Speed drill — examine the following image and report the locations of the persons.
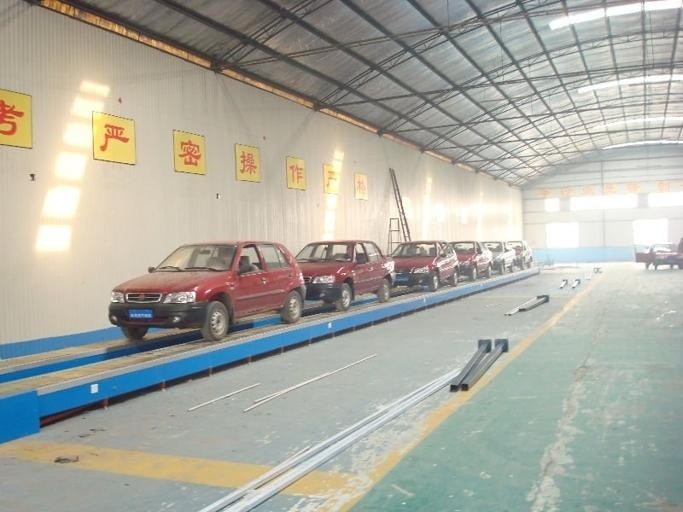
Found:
[678,238,683,269]
[221,247,249,273]
[346,245,364,266]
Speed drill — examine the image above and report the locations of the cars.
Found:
[627,239,682,270]
[382,238,459,293]
[104,237,308,343]
[481,241,517,275]
[445,239,495,283]
[506,239,534,270]
[292,240,397,313]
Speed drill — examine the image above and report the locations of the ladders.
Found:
[389,168,411,242]
